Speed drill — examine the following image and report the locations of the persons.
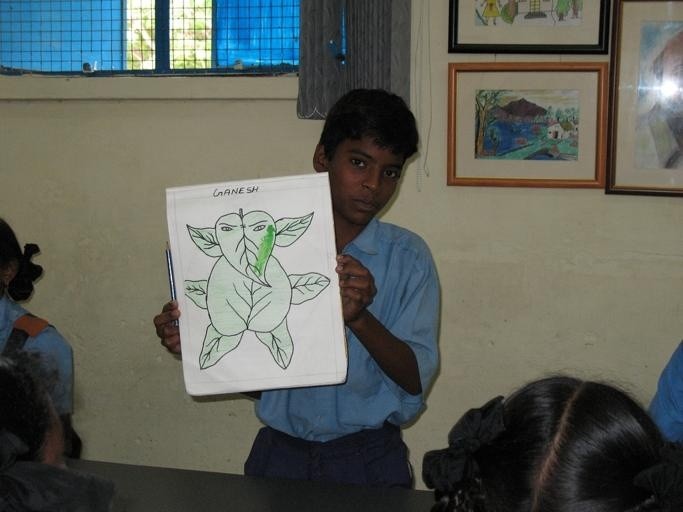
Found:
[0,213,75,469]
[421,370,682,512]
[649,27,682,170]
[0,349,118,512]
[151,85,445,491]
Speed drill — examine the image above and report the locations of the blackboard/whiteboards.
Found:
[166,172,348,396]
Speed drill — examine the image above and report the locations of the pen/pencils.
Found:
[165,242,179,328]
